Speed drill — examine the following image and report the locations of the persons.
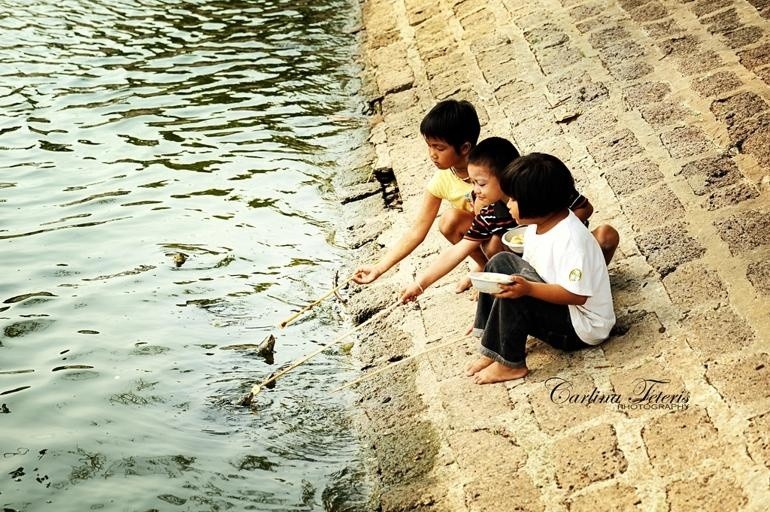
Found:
[464,153,618,386]
[397,133,621,306]
[352,99,491,295]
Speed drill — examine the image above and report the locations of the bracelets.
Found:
[414,279,424,294]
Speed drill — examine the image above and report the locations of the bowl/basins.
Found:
[500,225,530,254]
[466,272,515,295]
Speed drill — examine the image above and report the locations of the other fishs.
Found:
[256,334,275,354]
[230,392,253,406]
